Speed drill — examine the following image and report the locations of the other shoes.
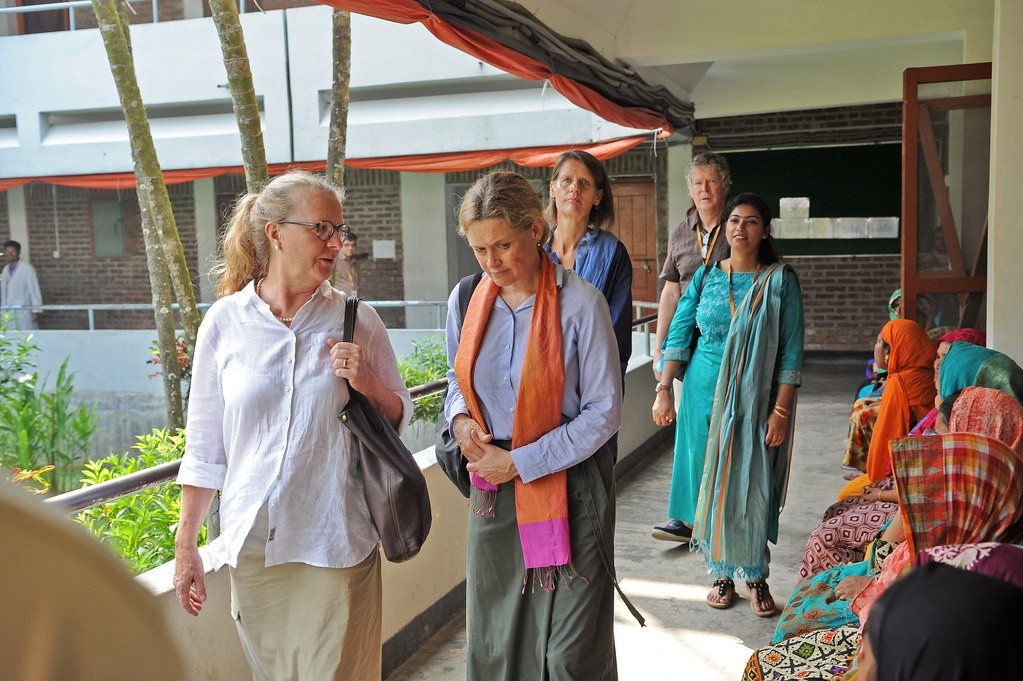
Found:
[845,472,860,480]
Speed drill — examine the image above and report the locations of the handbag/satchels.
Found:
[675,264,714,382]
[433,272,483,498]
[337,296,432,563]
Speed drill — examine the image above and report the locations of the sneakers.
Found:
[652,519,693,542]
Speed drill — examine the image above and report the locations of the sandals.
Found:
[707,577,733,607]
[746,580,778,615]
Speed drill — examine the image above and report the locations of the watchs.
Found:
[655,383,670,392]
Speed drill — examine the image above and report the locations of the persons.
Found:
[543,149,634,464]
[174,171,413,681]
[652,192,805,615]
[651,153,731,542]
[744,318,1023,681]
[333,233,358,297]
[0,240,43,330]
[443,172,622,681]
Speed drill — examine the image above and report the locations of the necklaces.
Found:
[257,277,292,322]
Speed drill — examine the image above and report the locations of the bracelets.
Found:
[774,405,790,418]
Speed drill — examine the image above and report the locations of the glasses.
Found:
[276,221,352,243]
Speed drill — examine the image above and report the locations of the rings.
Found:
[344,359,347,367]
